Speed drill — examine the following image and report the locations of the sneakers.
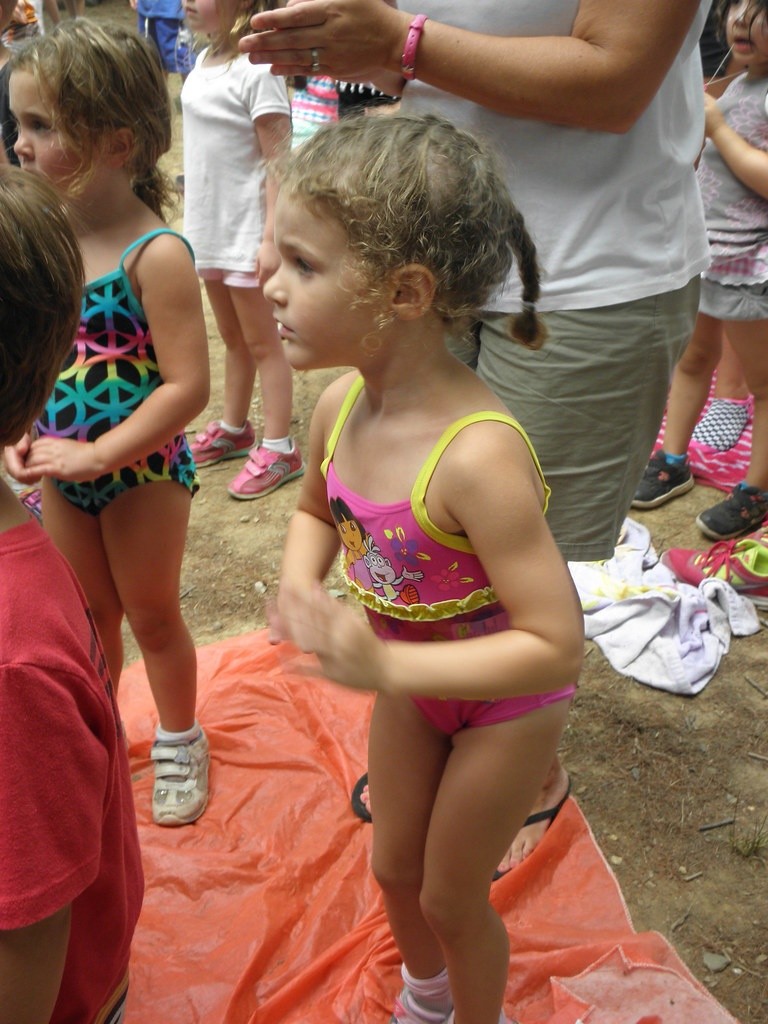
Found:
[228,436,305,501]
[150,732,211,824]
[189,420,255,468]
[659,538,768,610]
[629,450,695,508]
[696,482,768,539]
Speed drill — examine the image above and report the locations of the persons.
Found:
[0,18,209,828]
[0,0,768,880]
[263,116,587,1024]
[0,160,146,1024]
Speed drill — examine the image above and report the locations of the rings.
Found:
[307,47,323,71]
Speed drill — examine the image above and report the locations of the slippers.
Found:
[489,775,572,884]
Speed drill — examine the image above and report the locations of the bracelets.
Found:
[402,12,431,80]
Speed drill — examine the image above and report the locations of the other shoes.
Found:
[350,770,372,822]
